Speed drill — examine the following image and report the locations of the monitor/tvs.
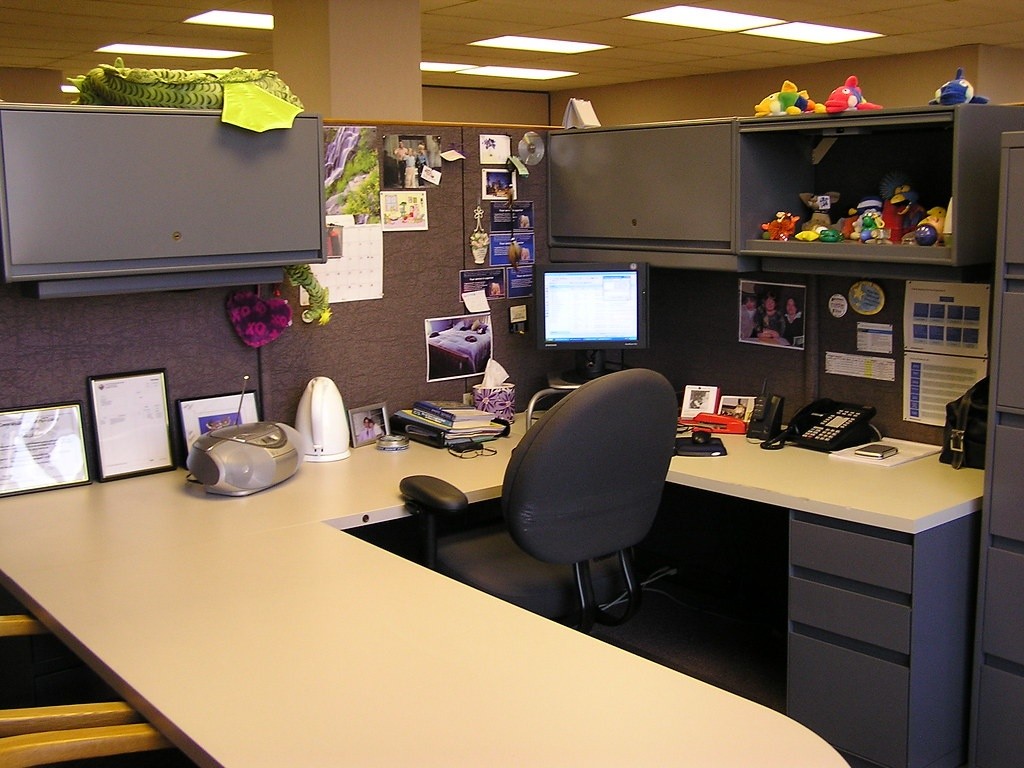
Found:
[534,261,651,389]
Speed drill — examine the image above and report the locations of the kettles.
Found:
[294,376,351,462]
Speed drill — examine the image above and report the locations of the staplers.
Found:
[693,412,749,435]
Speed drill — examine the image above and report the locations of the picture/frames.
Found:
[87,367,179,483]
[175,389,263,471]
[348,403,394,448]
[0,400,94,499]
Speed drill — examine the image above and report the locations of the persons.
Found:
[416,141,430,186]
[783,297,803,347]
[488,182,501,194]
[399,202,407,218]
[403,147,417,188]
[328,224,341,255]
[358,417,377,444]
[394,137,407,185]
[749,296,782,339]
[368,419,385,437]
[504,185,510,195]
[389,206,416,223]
[732,399,746,418]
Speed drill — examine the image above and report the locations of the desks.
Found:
[0,408,985,768]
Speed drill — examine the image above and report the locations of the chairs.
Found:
[400,368,688,631]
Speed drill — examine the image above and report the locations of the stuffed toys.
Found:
[754,80,824,117]
[824,75,883,113]
[926,65,988,108]
[66,52,304,134]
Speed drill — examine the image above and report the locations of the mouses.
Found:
[691,430,711,443]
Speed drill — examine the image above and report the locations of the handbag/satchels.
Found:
[940,376,989,470]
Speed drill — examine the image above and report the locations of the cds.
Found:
[519,131,544,165]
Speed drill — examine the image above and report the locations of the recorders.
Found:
[187,375,306,497]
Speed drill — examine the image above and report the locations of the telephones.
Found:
[785,397,877,451]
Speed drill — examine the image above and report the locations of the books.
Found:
[832,436,943,467]
[394,399,506,433]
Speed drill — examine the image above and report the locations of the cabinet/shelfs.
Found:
[788,515,979,768]
[549,102,1022,274]
[1,113,326,302]
[932,132,1024,768]
